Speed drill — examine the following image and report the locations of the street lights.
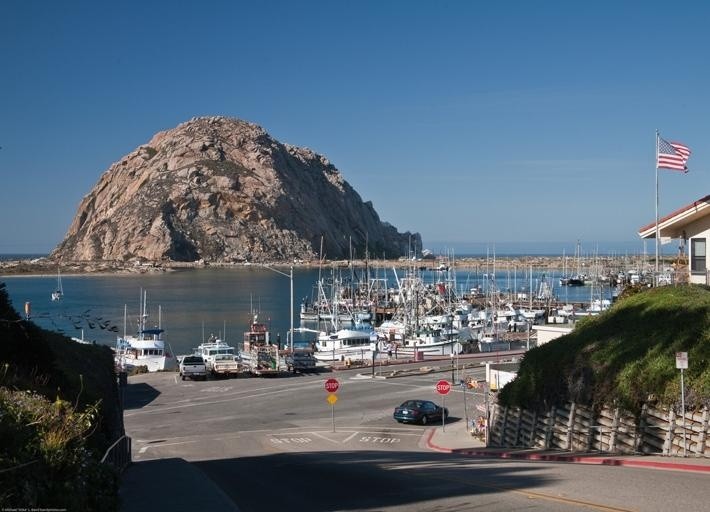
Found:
[244,262,295,353]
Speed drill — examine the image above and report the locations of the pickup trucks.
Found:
[285,351,319,374]
[179,355,207,380]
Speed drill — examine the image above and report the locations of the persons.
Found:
[383,334,398,360]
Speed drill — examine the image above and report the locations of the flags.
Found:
[657,136,691,174]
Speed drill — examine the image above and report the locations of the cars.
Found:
[394,400,448,425]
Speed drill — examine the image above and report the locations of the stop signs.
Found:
[435,380,451,396]
[325,378,339,393]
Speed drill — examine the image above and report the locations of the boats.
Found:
[71,288,178,373]
[196,294,290,380]
[52,267,64,301]
